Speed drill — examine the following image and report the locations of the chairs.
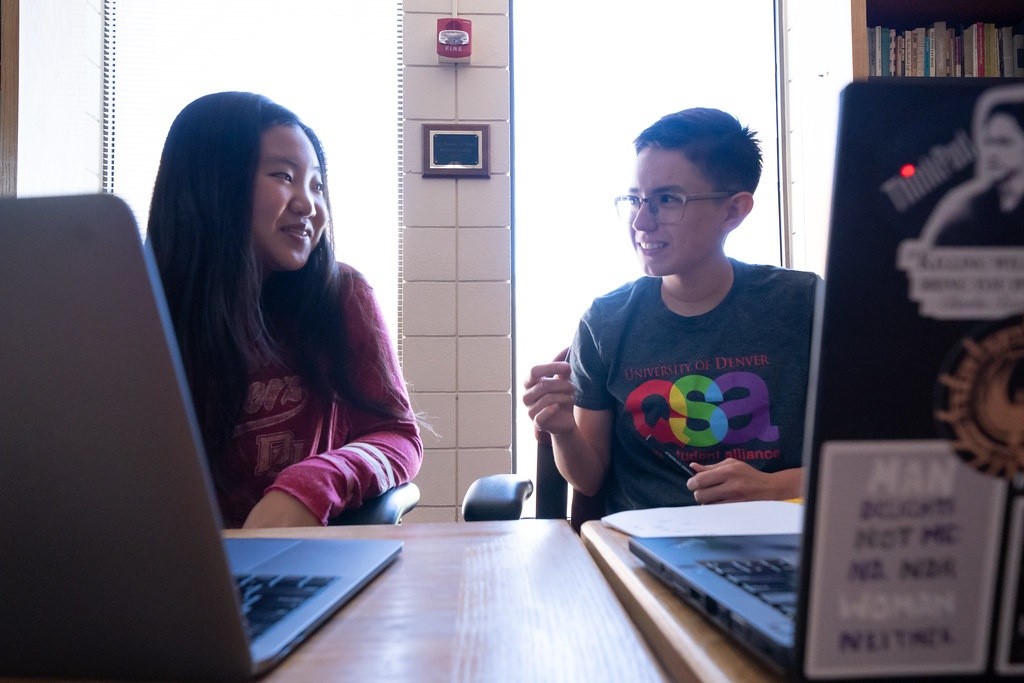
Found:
[462,347,572,520]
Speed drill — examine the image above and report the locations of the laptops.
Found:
[627,77,1024,683]
[2,192,406,683]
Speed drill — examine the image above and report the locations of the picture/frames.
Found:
[421,123,491,179]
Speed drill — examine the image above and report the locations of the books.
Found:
[866,22,1024,78]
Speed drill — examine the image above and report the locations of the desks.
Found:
[222,518,788,682]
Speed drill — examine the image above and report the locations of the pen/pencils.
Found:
[645,433,698,478]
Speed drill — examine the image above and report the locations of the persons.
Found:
[142,89,423,533]
[524,108,818,522]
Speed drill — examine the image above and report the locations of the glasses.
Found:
[614,191,730,224]
